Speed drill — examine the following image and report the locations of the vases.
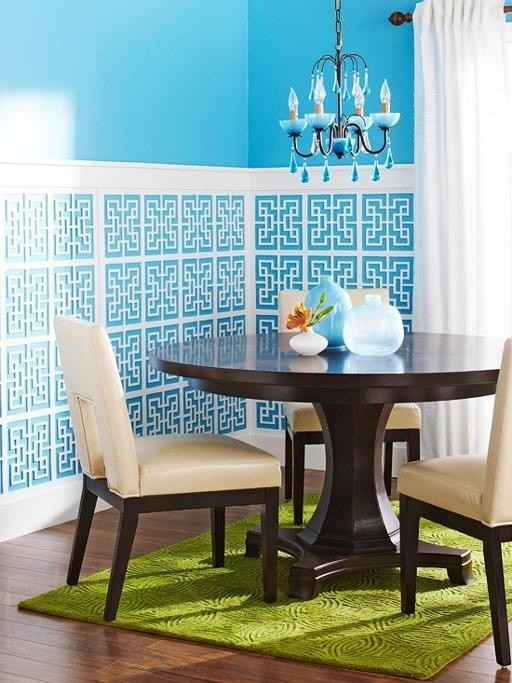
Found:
[304,275,352,346]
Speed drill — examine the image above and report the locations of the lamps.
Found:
[277,0,402,183]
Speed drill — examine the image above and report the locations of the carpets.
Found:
[18,489,511,680]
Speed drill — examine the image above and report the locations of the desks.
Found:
[148,330,510,603]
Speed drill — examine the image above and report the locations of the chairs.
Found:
[50,286,511,666]
